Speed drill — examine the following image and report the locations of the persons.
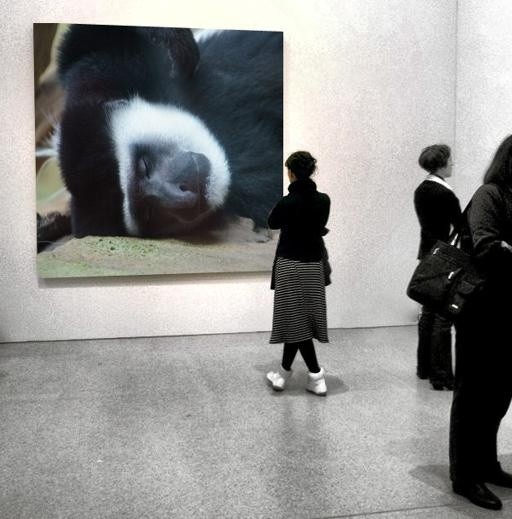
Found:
[413,143,462,392]
[448,134,512,512]
[265,149,331,397]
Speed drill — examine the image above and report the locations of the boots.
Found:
[305,367,328,396]
[265,364,294,392]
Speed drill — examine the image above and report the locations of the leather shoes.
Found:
[452,479,503,511]
[416,367,459,390]
[480,461,512,489]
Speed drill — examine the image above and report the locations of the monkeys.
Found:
[33,24,283,255]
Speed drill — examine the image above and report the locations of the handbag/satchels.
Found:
[407,240,483,325]
